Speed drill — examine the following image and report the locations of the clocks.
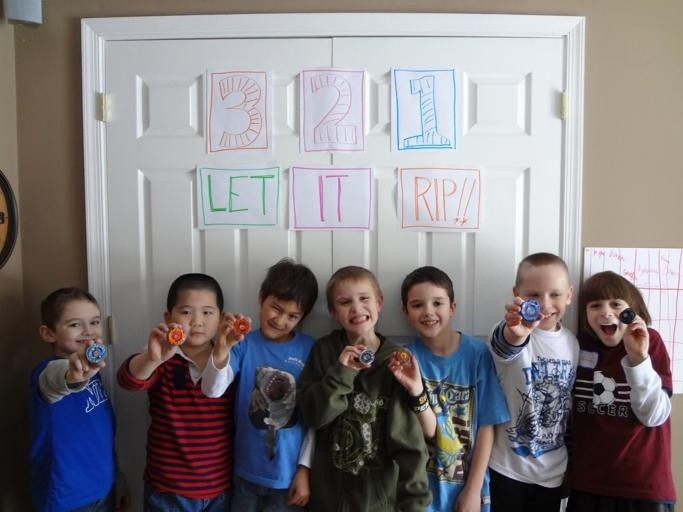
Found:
[0,169,19,269]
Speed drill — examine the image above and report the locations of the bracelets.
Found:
[410,388,430,413]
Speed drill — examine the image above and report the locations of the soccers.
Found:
[592,371,618,410]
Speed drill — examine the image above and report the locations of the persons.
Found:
[201,257,318,512]
[296,266,432,512]
[117,274,235,512]
[565,271,676,512]
[388,267,512,512]
[27,286,115,512]
[486,253,580,512]
